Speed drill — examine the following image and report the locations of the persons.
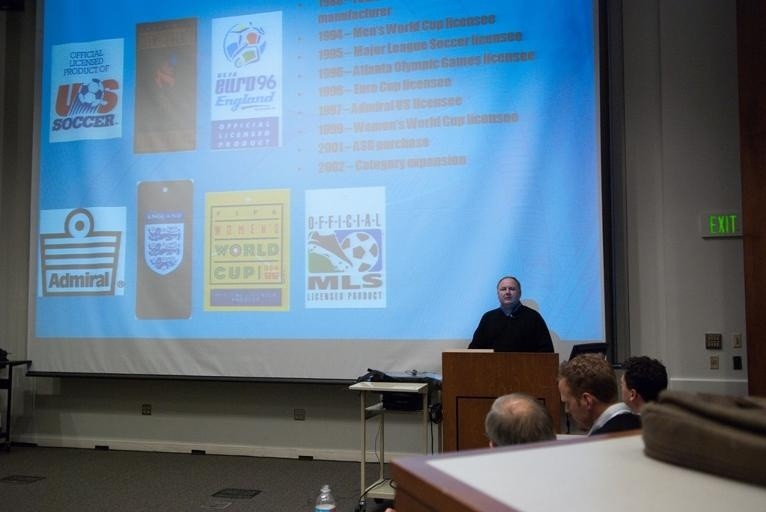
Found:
[619,355,668,417]
[484,392,555,448]
[557,354,641,437]
[467,277,555,353]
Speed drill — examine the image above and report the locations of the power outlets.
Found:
[710,356,719,369]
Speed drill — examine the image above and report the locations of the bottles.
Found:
[314,484,336,512]
